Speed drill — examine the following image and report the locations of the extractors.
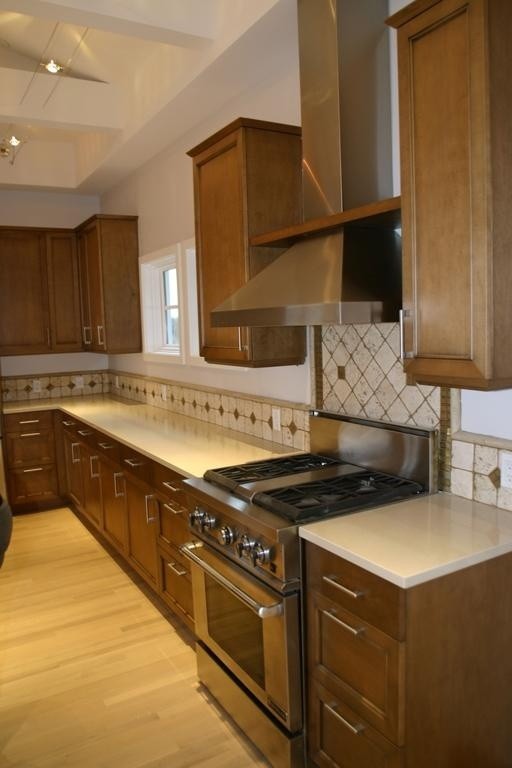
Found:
[207,0,405,328]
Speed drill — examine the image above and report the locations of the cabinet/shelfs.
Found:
[39,221,85,355]
[183,112,310,371]
[0,217,48,358]
[51,402,197,655]
[2,409,61,513]
[295,540,512,768]
[383,0,510,394]
[74,207,144,363]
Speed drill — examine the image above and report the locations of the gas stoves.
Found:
[183,453,423,583]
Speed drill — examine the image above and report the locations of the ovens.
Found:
[176,532,306,768]
